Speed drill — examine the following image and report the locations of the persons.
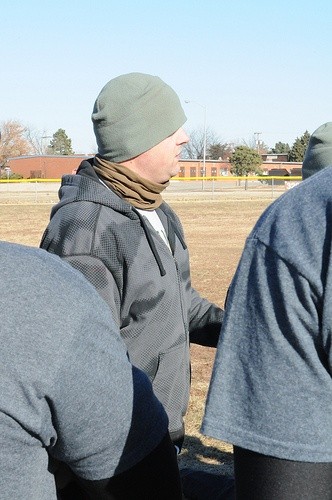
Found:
[0,240,181,500]
[202,161,332,500]
[35,71,232,499]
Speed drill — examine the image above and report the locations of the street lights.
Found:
[185,96,218,189]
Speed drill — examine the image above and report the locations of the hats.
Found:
[301,120,332,180]
[92,73,187,163]
